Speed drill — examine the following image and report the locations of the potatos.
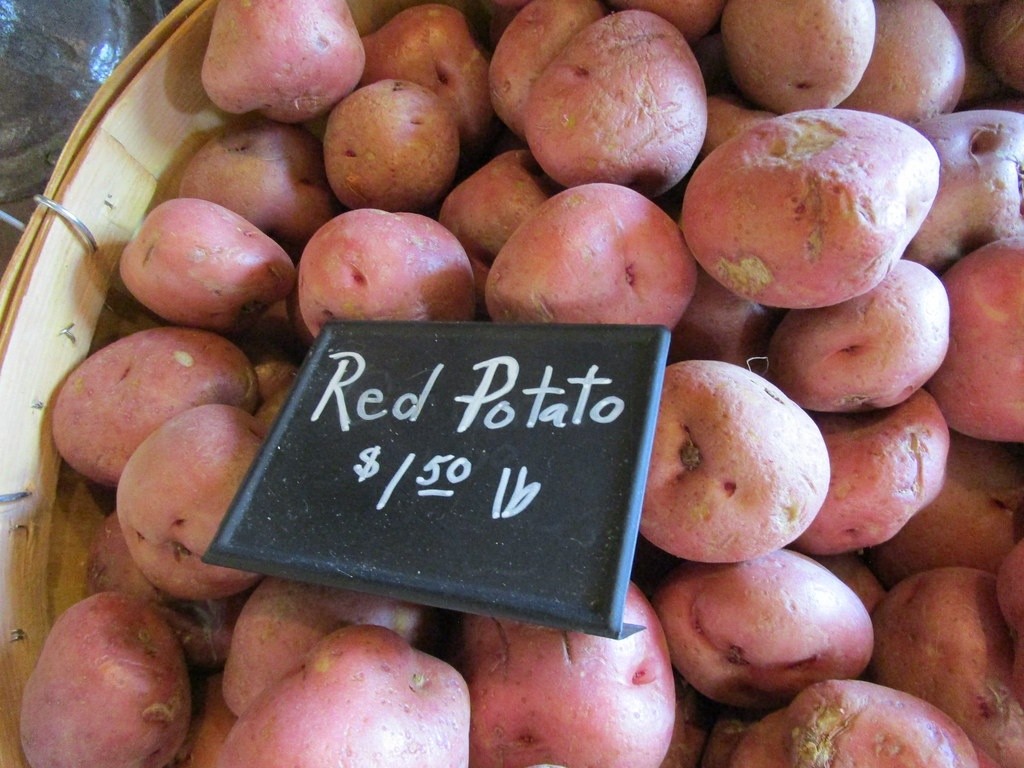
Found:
[20,0,1024,768]
